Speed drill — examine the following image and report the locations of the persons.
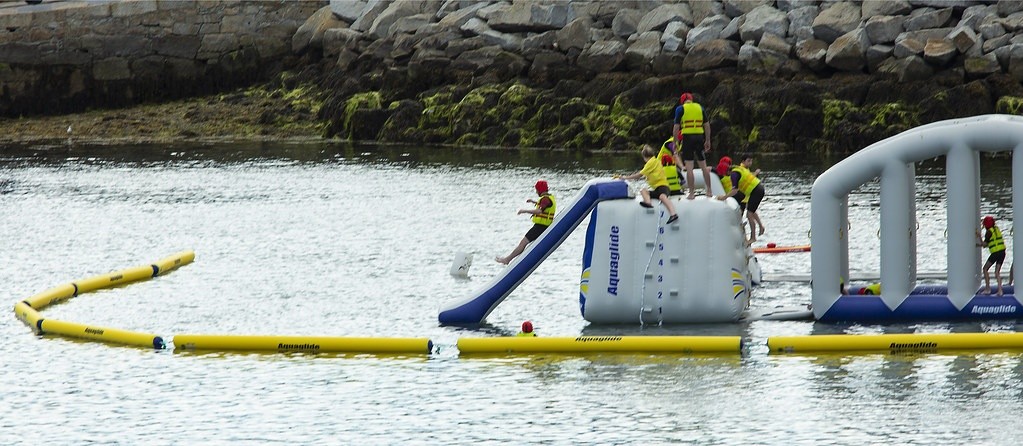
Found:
[672,92,714,200]
[494,179,555,264]
[806,276,850,310]
[657,129,687,184]
[718,154,767,245]
[618,145,678,225]
[1003,224,1014,287]
[979,216,1005,297]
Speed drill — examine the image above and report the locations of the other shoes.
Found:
[640,202,653,208]
[666,214,678,224]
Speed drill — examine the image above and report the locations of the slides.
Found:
[438,178,601,326]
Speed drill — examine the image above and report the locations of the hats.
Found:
[720,158,733,169]
[536,179,548,195]
[681,93,693,104]
[716,165,727,176]
[522,322,533,333]
[661,154,674,165]
[677,130,682,144]
[983,216,996,230]
